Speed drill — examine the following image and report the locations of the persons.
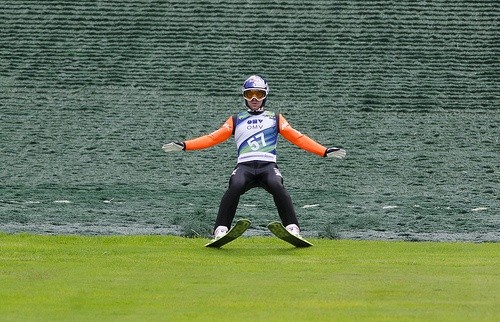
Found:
[161,75,348,248]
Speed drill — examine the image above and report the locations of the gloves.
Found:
[161,137,184,152]
[326,147,347,160]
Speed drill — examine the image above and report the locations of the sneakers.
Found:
[214,226,229,240]
[286,224,300,237]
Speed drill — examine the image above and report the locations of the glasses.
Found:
[243,89,267,101]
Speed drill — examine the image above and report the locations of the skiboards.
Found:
[206,220,316,247]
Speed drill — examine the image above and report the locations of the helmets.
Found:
[241,75,270,92]
